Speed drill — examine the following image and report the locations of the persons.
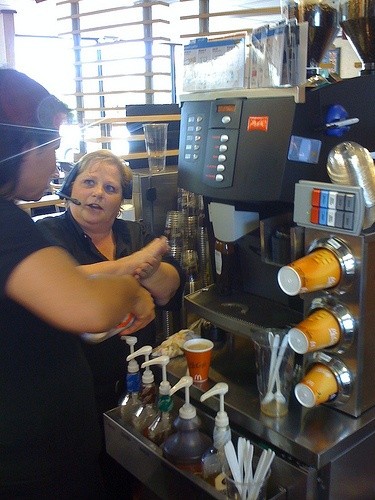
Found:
[0,68,155,500]
[34,149,186,500]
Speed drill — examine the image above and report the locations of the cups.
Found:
[295,363,340,408]
[277,248,340,296]
[222,457,272,500]
[156,187,215,343]
[288,308,340,355]
[143,123,168,172]
[183,339,214,385]
[252,327,303,419]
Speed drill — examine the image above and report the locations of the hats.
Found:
[0,69,71,136]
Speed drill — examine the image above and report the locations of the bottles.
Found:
[215,240,239,287]
